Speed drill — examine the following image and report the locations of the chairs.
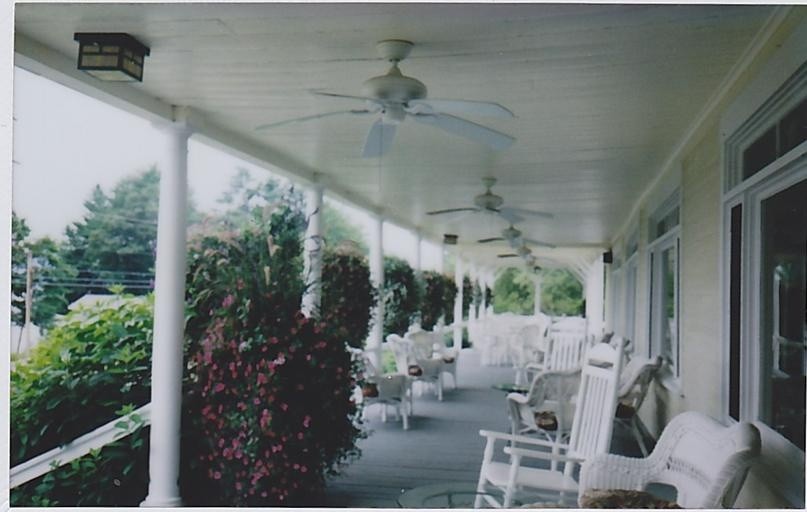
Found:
[343,324,459,432]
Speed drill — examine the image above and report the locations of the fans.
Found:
[415,171,558,269]
[252,35,517,162]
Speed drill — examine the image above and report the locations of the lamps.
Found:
[68,31,151,85]
